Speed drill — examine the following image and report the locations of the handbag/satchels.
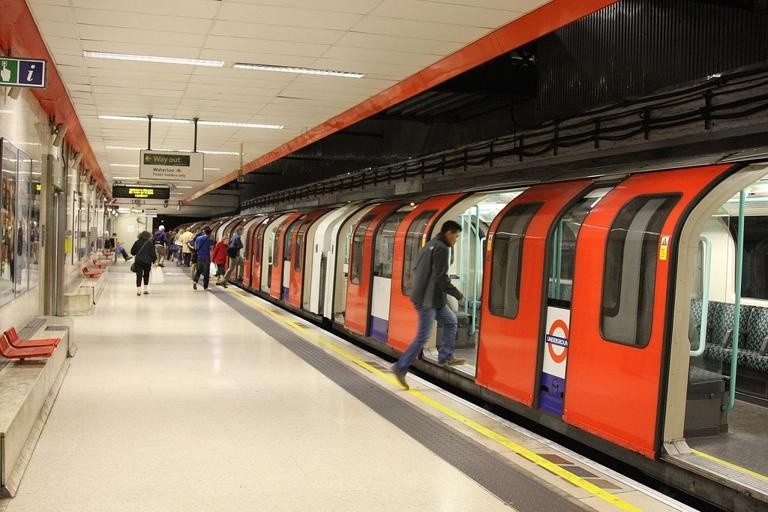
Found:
[191,252,198,264]
[227,247,237,259]
[151,265,164,284]
[130,262,137,272]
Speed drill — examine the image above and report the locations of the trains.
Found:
[170,157,767,510]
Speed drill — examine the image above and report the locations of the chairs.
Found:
[80,249,114,278]
[0,334,54,364]
[3,327,61,348]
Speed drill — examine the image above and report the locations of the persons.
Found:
[211,235,230,286]
[129,230,158,295]
[109,232,133,262]
[153,224,217,291]
[222,227,245,288]
[389,220,466,391]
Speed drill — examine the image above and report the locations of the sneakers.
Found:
[193,281,197,290]
[204,287,212,291]
[390,364,410,390]
[143,290,149,294]
[438,357,466,366]
[136,291,142,296]
